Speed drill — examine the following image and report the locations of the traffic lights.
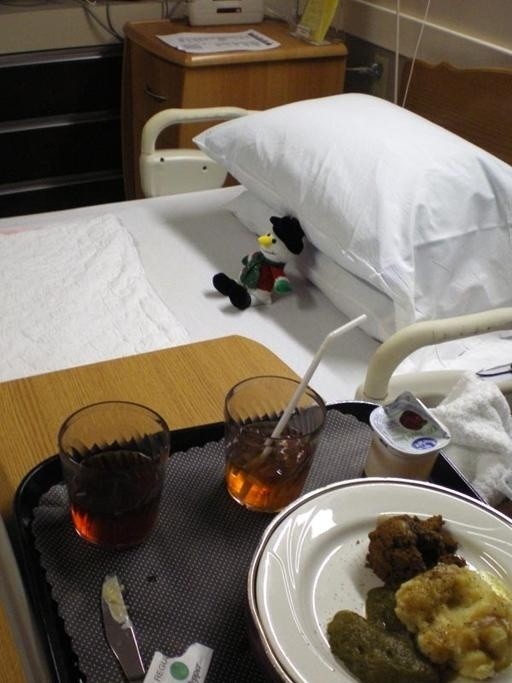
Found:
[222,189,415,344]
[193,94,512,329]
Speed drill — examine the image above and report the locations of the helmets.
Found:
[244,476,512,683]
[12,396,474,682]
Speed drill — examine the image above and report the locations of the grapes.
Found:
[115,19,349,203]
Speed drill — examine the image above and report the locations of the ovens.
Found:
[0,59,512,435]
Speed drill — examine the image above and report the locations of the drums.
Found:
[101,573,147,683]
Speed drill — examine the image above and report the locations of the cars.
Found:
[221,375,327,515]
[56,400,170,553]
